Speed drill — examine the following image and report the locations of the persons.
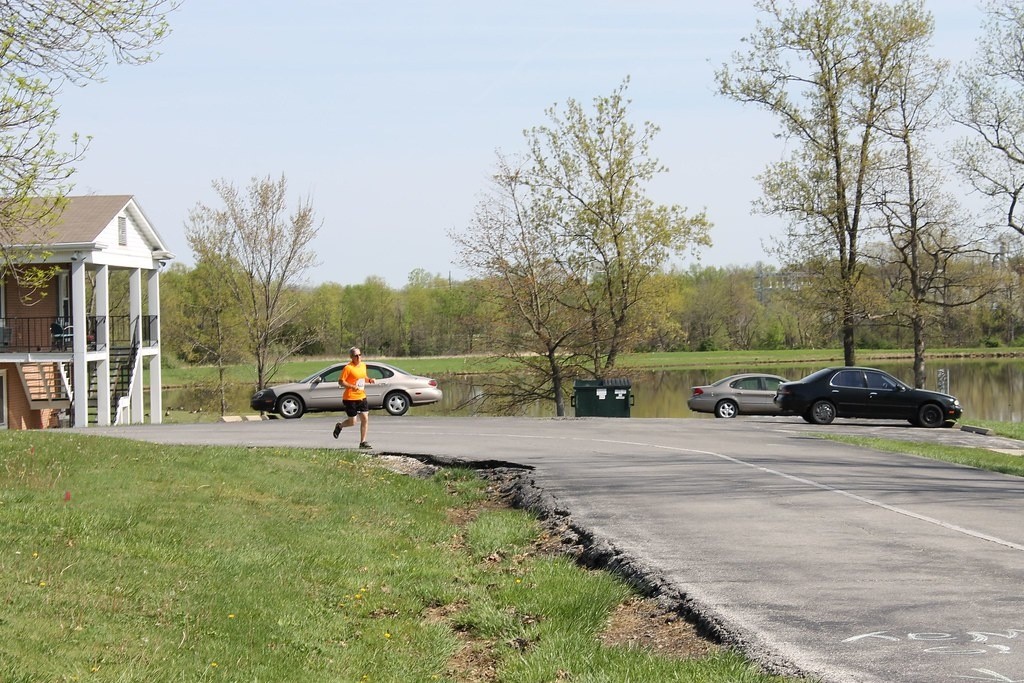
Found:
[332,347,376,449]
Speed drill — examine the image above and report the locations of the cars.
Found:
[251,362,443,418]
[687,373,801,418]
[773,366,963,428]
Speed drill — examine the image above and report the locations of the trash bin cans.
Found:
[571,379,634,418]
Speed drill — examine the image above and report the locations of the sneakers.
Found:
[359,441,372,449]
[333,423,342,439]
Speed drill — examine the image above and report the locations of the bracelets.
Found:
[369,379,372,384]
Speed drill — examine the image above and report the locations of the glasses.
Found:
[353,354,361,357]
